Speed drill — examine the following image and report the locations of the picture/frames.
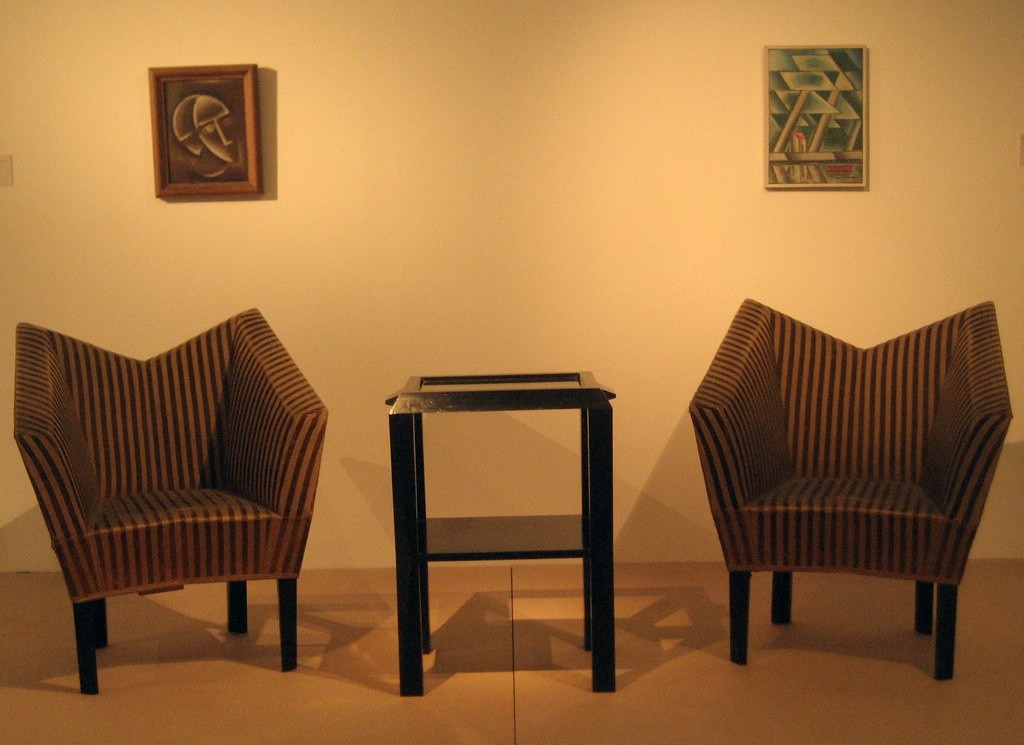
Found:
[764,45,870,190]
[149,63,263,194]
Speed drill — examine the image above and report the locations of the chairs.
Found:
[14,309,328,695]
[689,300,1014,680]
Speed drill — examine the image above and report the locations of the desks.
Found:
[384,372,617,696]
